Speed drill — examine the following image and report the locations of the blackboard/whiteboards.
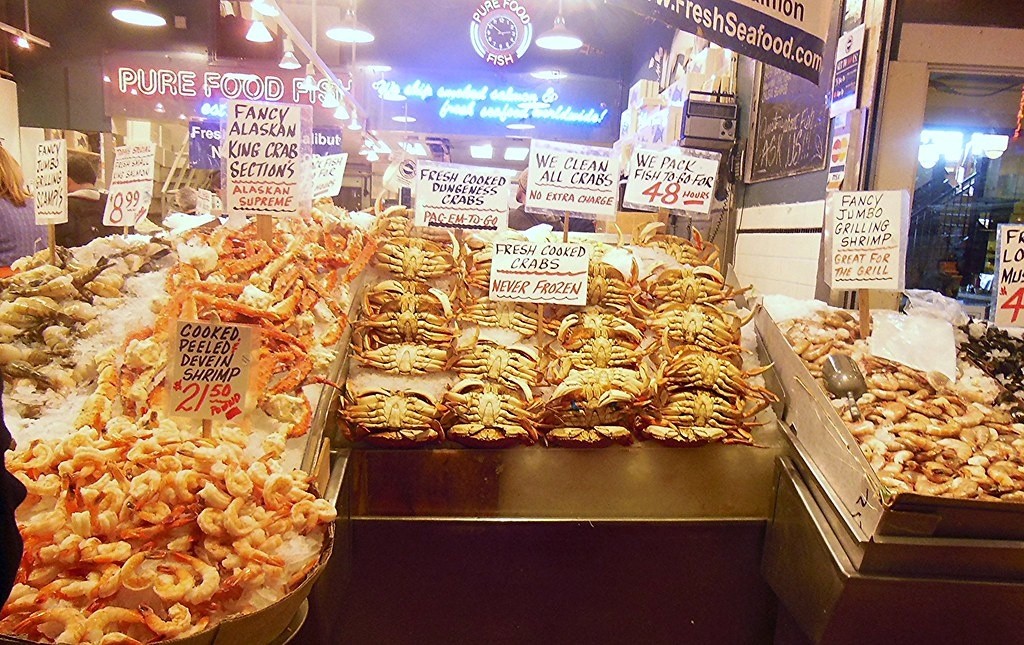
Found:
[752,0,846,181]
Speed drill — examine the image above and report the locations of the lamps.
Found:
[245,9,274,43]
[333,99,351,120]
[250,0,280,18]
[110,0,167,28]
[278,36,301,69]
[358,137,380,162]
[347,109,362,131]
[321,81,338,107]
[535,0,582,51]
[325,0,375,44]
[299,60,320,91]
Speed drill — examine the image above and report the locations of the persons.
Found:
[931,154,951,202]
[164,187,211,215]
[210,171,226,217]
[0,144,50,265]
[56,155,134,247]
[507,166,595,232]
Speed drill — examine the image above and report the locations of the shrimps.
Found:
[0,430,333,643]
[0,220,168,431]
[788,289,1023,507]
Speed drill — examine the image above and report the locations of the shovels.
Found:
[822,353,867,419]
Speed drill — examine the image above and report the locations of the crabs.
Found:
[79,203,778,449]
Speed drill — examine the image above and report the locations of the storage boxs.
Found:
[612,48,729,172]
